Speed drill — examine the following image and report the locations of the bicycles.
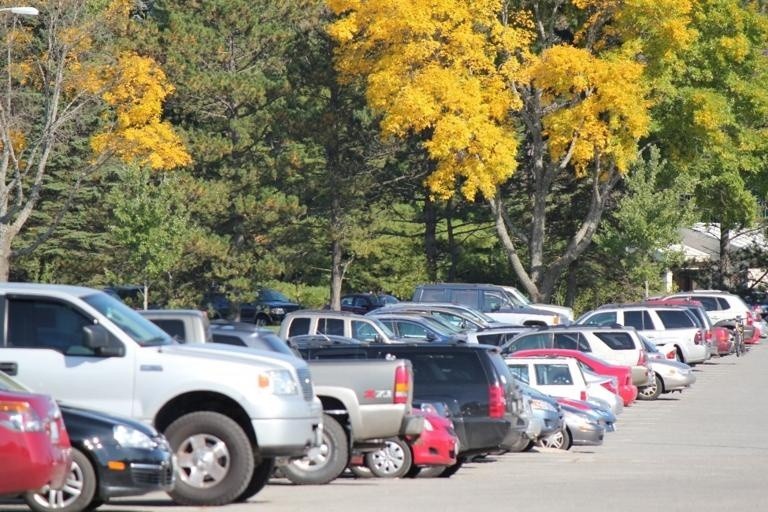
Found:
[723,313,752,358]
